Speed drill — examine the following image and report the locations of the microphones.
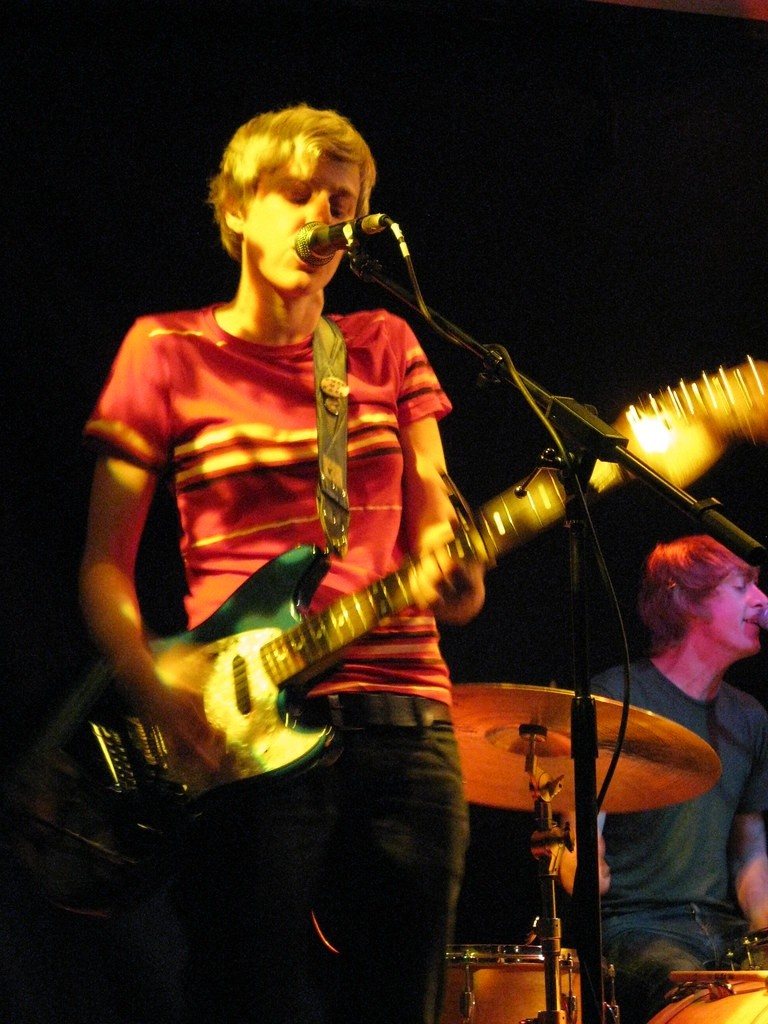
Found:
[294,212,392,267]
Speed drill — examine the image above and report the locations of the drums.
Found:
[719,927,768,970]
[646,979,768,1024]
[435,939,587,1023]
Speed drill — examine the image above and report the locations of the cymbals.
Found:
[448,680,724,816]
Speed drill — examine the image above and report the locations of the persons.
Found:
[76,102,488,1023]
[578,528,768,1024]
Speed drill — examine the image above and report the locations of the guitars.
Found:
[62,357,768,864]
